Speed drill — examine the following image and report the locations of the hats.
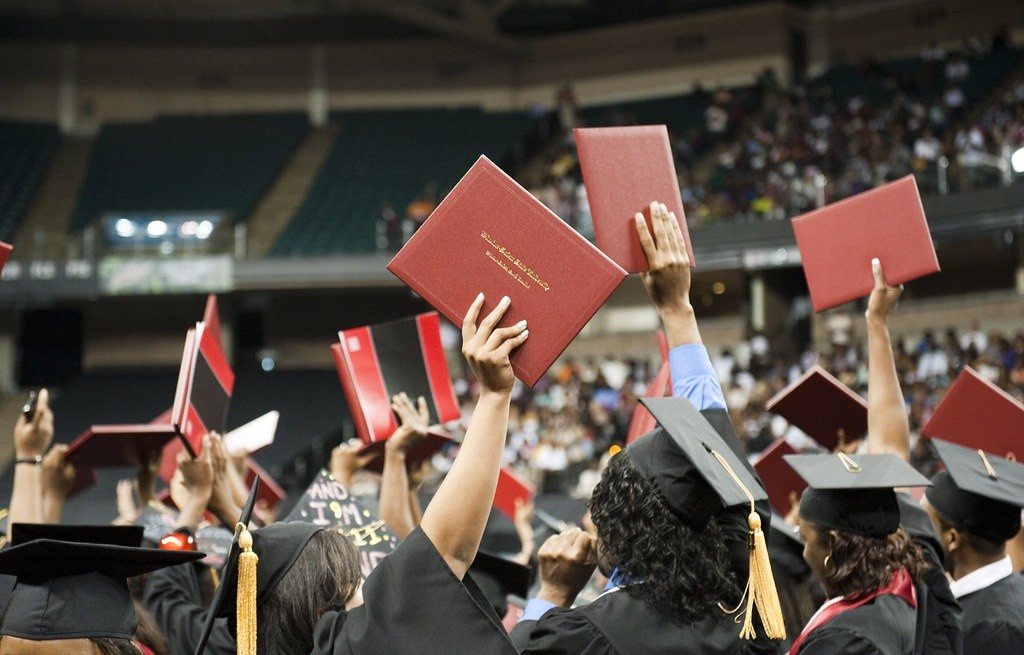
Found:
[770,453,935,584]
[926,437,1024,540]
[468,549,532,616]
[0,473,329,655]
[626,397,787,640]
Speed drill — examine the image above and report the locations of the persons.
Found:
[0,306,1024,578]
[926,436,1024,654]
[0,537,206,655]
[526,27,1024,235]
[782,257,961,653]
[142,290,530,654]
[511,199,786,655]
